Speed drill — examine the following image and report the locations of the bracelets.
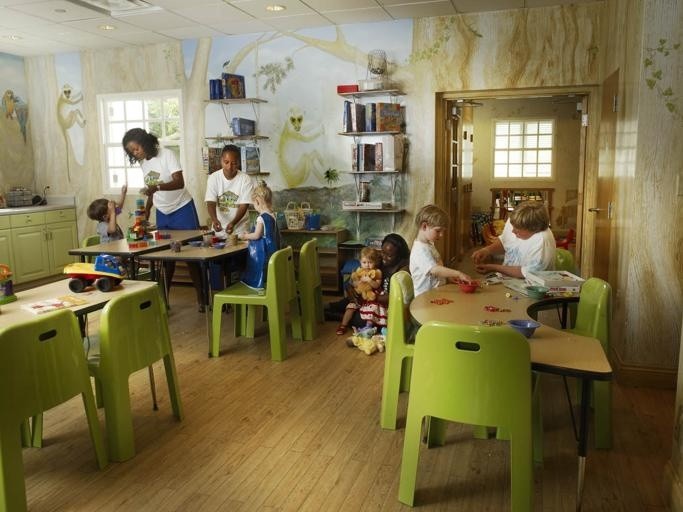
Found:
[156,185,160,190]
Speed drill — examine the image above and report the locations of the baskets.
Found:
[284,201,321,230]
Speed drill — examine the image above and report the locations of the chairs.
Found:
[202,237,322,362]
[560,189,578,229]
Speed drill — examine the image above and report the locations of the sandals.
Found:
[335,324,347,335]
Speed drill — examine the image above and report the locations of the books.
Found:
[341,98,403,172]
[341,201,392,210]
[19,294,89,315]
[199,73,259,173]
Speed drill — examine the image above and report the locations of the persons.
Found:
[408,204,472,297]
[86,184,126,243]
[237,179,280,293]
[320,233,409,329]
[204,145,253,313]
[335,246,384,336]
[472,200,556,276]
[122,126,206,313]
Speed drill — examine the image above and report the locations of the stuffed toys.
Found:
[345,327,386,354]
[351,269,376,300]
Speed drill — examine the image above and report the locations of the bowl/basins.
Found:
[212,243,225,248]
[188,241,202,247]
[460,281,476,293]
[526,285,549,300]
[508,319,541,337]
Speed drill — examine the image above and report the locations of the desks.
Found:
[66,226,247,311]
[0,278,159,339]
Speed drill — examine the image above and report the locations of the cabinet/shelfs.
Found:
[11,209,78,286]
[336,87,405,252]
[488,185,555,226]
[0,216,12,285]
[202,98,270,178]
[277,226,350,295]
[445,116,474,268]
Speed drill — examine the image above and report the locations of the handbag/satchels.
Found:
[382,135,404,172]
